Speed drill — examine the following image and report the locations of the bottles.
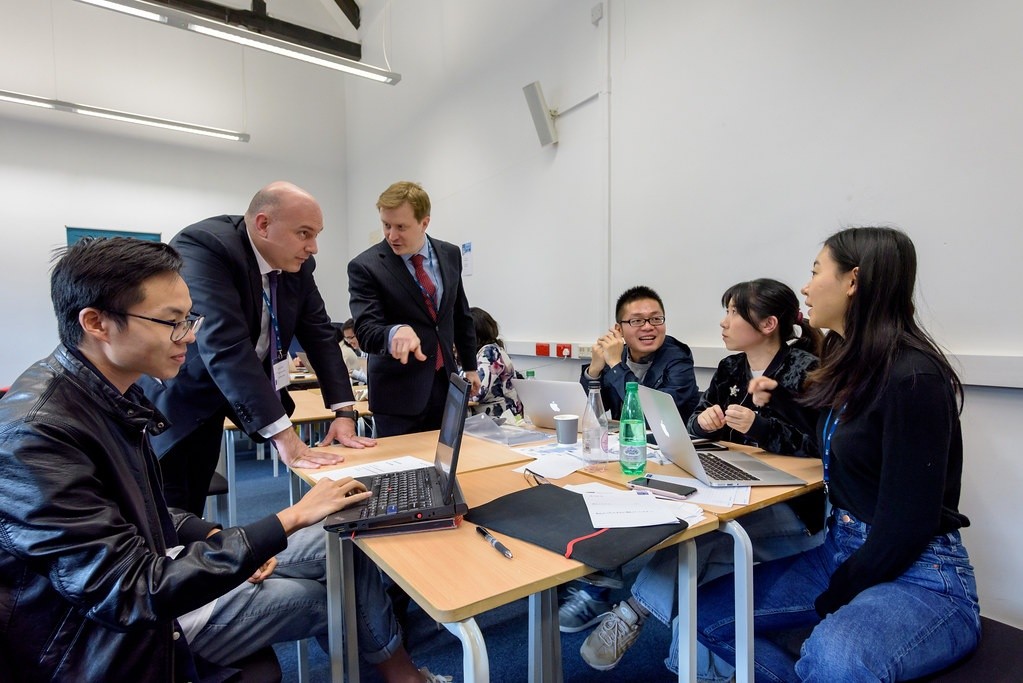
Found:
[582,381,608,473]
[523,370,537,425]
[619,382,647,476]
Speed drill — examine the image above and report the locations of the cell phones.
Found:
[627,477,697,500]
[694,443,728,450]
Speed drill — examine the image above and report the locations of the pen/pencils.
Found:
[476,526,513,558]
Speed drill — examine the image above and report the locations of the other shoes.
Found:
[420,666,453,683]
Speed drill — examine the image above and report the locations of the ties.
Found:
[411,255,443,370]
[268,270,281,402]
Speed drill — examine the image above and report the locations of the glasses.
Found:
[344,335,355,342]
[127,313,204,342]
[622,314,665,327]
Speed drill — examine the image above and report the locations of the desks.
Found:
[221,361,827,683]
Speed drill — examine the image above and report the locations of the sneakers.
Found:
[580,601,643,670]
[558,588,622,633]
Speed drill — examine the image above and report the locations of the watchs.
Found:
[335,410,359,421]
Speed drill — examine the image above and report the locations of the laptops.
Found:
[510,379,621,434]
[637,384,809,487]
[323,371,472,533]
[287,350,316,375]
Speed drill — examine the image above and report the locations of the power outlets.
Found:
[557,344,571,357]
[536,343,549,356]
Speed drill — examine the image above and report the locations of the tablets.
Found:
[646,432,711,449]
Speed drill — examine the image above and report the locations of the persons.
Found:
[454,307,525,420]
[0,236,452,683]
[581,278,827,683]
[348,181,481,440]
[135,181,377,519]
[697,227,981,683]
[559,285,700,632]
[343,315,373,384]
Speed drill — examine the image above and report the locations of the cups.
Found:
[554,415,579,447]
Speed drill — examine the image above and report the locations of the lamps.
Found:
[75,0,401,86]
[0,89,250,143]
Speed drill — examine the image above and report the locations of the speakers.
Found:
[522,81,558,146]
